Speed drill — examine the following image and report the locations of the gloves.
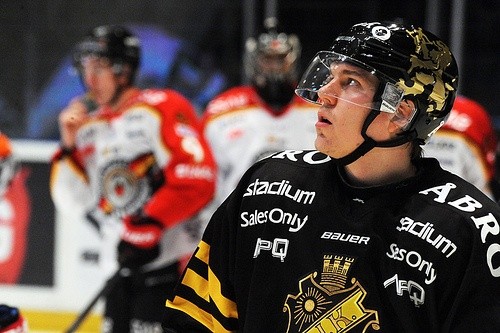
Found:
[117,207,164,270]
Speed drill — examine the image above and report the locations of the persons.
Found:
[160,20,500,333]
[50,24,217,333]
[201,29,321,279]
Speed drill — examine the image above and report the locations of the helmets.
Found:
[244,17,304,89]
[73,23,140,89]
[294,22,459,145]
